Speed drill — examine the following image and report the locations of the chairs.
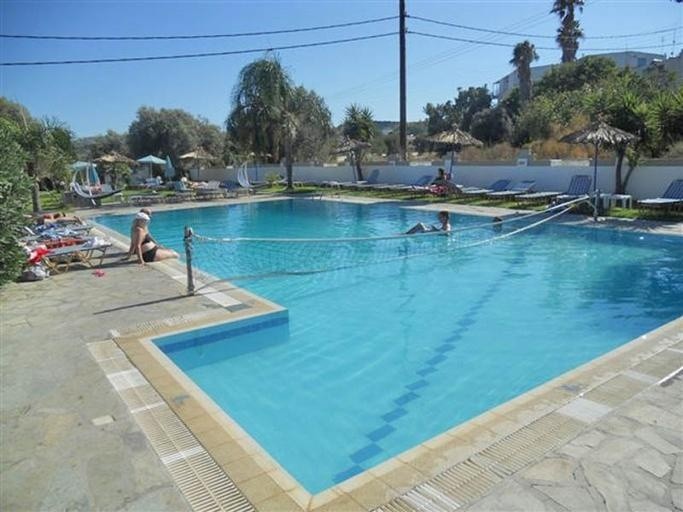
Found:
[312,168,683,218]
[68,160,301,206]
[13,209,113,274]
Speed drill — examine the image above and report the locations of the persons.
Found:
[129,208,167,253]
[411,166,445,190]
[406,210,450,236]
[492,218,502,228]
[126,212,181,266]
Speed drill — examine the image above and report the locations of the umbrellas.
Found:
[64,159,97,172]
[177,145,218,179]
[327,136,372,182]
[557,120,644,195]
[138,156,164,178]
[94,150,135,188]
[423,123,484,179]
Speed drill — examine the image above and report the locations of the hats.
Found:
[134,212,151,222]
